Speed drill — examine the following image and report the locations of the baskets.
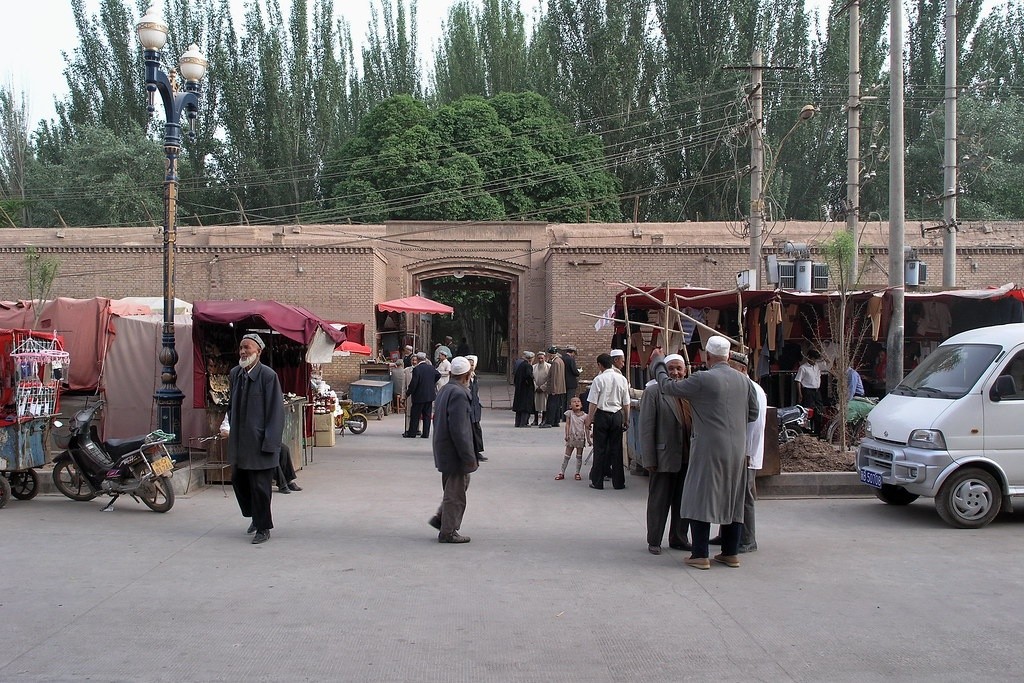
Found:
[52,425,73,449]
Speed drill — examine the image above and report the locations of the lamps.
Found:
[453,270,464,278]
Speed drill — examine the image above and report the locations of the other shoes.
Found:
[647,545,661,554]
[515,424,531,428]
[252,528,270,544]
[279,486,290,493]
[714,553,739,567]
[247,521,256,533]
[685,556,712,569]
[674,541,692,550]
[707,536,757,553]
[288,482,302,491]
[429,516,471,543]
[539,423,552,428]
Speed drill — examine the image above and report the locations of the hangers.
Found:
[42,331,71,363]
[9,328,47,362]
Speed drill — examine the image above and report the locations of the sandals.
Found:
[575,473,581,480]
[554,473,564,480]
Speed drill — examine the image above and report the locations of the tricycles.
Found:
[0,414,53,508]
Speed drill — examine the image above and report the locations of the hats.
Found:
[610,349,623,357]
[405,345,412,350]
[410,352,426,360]
[242,334,265,350]
[395,358,403,366]
[664,353,684,364]
[440,350,447,355]
[435,343,442,347]
[446,336,452,339]
[706,336,749,365]
[523,346,578,357]
[450,355,478,375]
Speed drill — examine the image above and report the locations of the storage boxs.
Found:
[314,414,336,446]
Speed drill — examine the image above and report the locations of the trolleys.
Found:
[349,378,394,420]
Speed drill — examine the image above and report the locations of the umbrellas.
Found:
[377,294,453,356]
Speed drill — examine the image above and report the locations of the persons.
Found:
[272,442,303,494]
[640,353,693,553]
[586,353,630,489]
[401,335,470,437]
[709,350,768,551]
[589,349,625,478]
[842,362,864,398]
[651,336,759,570]
[512,344,581,428]
[429,355,478,543]
[795,350,823,434]
[465,355,488,460]
[555,397,592,479]
[874,348,887,378]
[226,333,286,544]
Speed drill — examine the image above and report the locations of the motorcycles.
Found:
[776,404,808,444]
[52,400,176,512]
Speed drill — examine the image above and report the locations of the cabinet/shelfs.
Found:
[184,436,229,497]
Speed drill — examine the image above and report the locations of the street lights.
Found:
[136,5,207,462]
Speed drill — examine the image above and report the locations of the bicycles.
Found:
[334,400,367,434]
[827,396,879,451]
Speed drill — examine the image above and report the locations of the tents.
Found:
[614,284,1024,365]
[0,296,370,455]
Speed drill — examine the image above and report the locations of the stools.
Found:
[392,395,406,414]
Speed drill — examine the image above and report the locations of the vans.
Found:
[854,323,1024,530]
